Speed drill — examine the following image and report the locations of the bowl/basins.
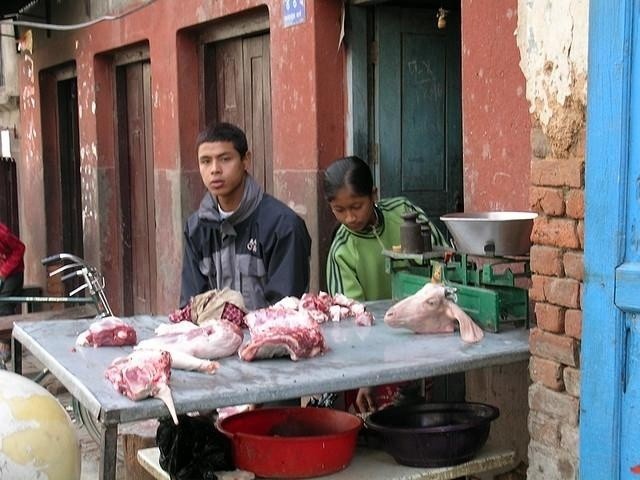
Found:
[364,400,501,468]
[438,209,539,257]
[214,406,363,479]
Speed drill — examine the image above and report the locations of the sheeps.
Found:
[384,282,484,343]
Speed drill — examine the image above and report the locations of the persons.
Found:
[0,222,25,363]
[178,124,312,407]
[322,156,451,415]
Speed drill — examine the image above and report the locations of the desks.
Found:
[10,299,537,480]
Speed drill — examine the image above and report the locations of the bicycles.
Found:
[1,254,126,462]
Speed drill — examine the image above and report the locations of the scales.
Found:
[381,212,537,334]
